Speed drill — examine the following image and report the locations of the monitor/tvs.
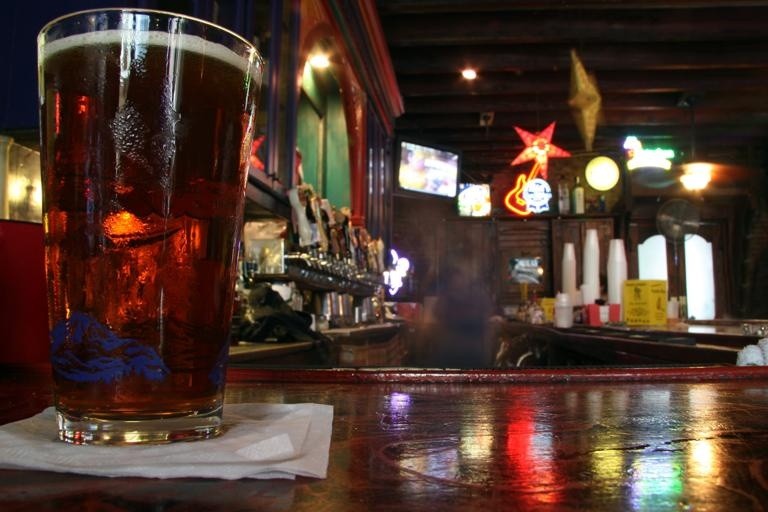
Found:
[395,139,457,197]
[383,241,417,302]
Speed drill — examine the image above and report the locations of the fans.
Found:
[655,196,702,319]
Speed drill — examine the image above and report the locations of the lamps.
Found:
[674,90,721,194]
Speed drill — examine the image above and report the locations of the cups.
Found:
[558,227,629,327]
[324,290,378,325]
[36,8,262,448]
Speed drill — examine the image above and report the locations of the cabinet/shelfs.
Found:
[232,1,298,198]
[552,214,614,300]
[619,195,734,323]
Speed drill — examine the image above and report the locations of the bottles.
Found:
[555,293,575,329]
[557,174,574,215]
[571,176,589,215]
